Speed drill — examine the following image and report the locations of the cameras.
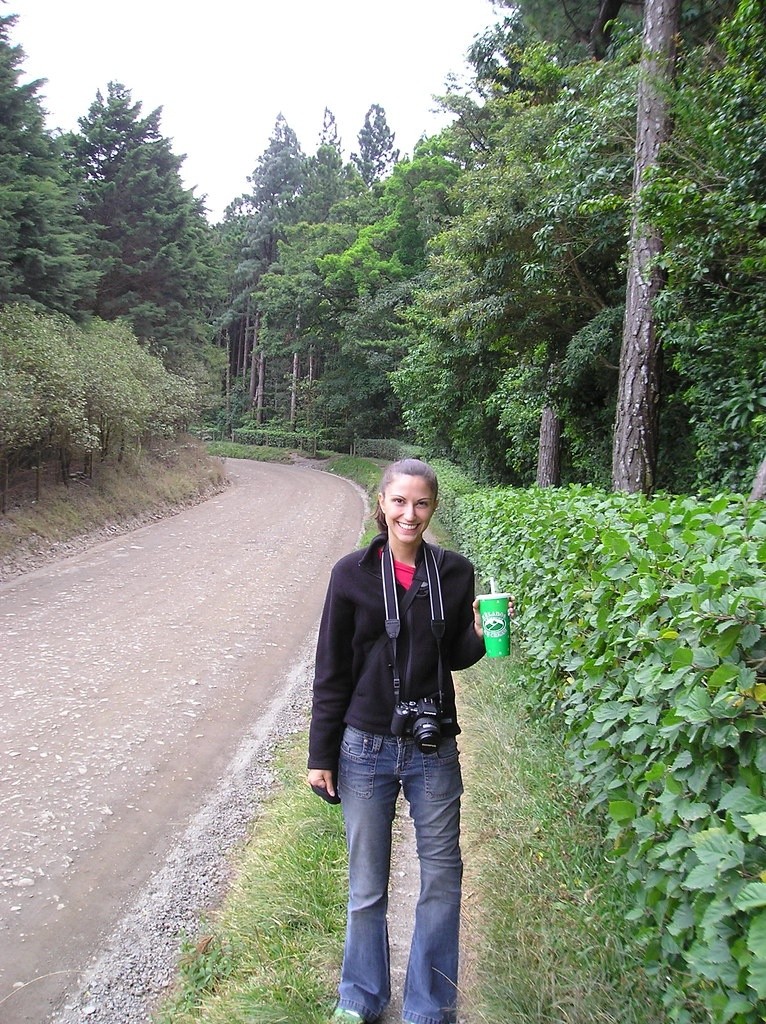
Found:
[391,698,443,755]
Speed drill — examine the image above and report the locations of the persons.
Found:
[307,459,516,1024]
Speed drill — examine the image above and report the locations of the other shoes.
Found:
[328,1006,365,1024]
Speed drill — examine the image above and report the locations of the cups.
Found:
[478,593,511,658]
